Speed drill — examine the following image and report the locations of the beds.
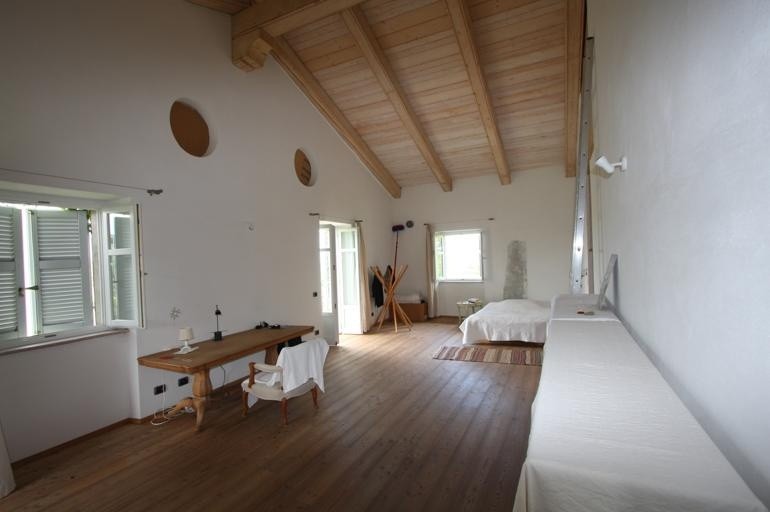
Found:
[464,295,551,348]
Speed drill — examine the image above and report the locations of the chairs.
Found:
[240,337,327,426]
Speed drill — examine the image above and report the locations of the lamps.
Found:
[594,152,628,176]
[211,304,227,341]
[173,327,199,357]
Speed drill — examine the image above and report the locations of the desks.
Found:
[549,294,618,322]
[522,367,768,512]
[456,300,483,323]
[543,321,655,367]
[138,324,314,434]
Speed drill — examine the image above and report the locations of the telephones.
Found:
[468,298,478,303]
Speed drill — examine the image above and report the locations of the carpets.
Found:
[433,343,543,368]
